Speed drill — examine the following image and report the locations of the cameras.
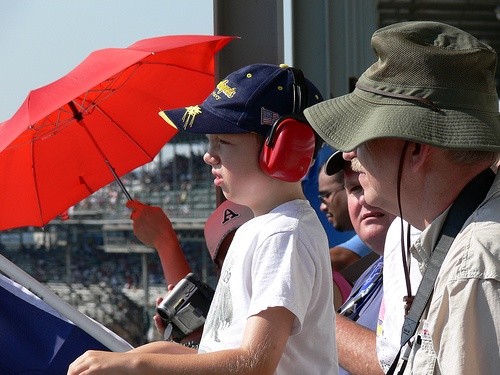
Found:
[156,273,216,340]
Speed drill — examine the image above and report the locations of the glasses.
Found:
[318,185,342,204]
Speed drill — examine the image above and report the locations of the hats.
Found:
[204,201,254,264]
[302,22,500,153]
[323,150,347,175]
[159,64,326,158]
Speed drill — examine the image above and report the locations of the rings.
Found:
[129,210,135,220]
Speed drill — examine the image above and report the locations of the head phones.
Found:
[257,68,317,184]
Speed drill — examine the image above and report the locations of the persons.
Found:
[342,20,500,375]
[64,63,338,375]
[125,145,397,375]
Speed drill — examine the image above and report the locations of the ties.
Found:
[340,258,383,316]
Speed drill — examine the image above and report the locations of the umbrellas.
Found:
[0,34,242,233]
